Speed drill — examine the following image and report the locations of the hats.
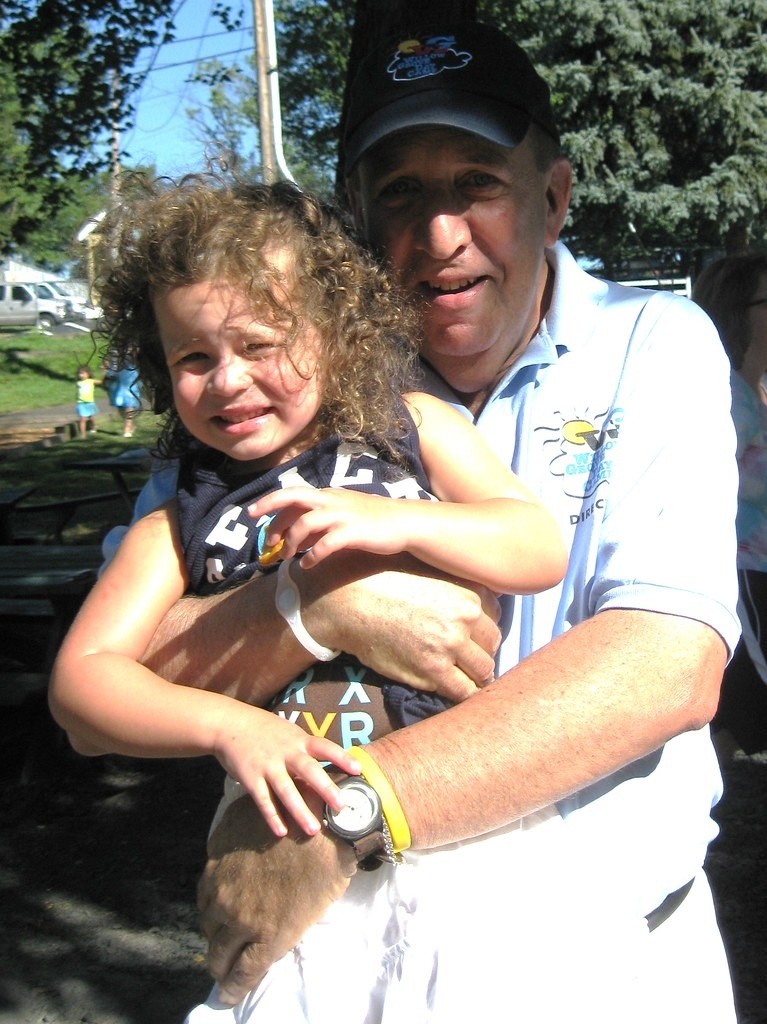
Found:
[342,15,560,177]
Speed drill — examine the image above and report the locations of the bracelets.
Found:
[275,559,341,661]
[381,816,405,866]
[347,745,413,853]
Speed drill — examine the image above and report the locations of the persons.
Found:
[48,174,687,1024]
[50,10,740,1024]
[693,252,767,768]
[102,340,143,439]
[71,361,107,441]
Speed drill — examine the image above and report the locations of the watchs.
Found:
[321,764,387,872]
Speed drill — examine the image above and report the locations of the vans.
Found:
[0,278,107,332]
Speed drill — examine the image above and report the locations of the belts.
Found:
[645,878,695,934]
